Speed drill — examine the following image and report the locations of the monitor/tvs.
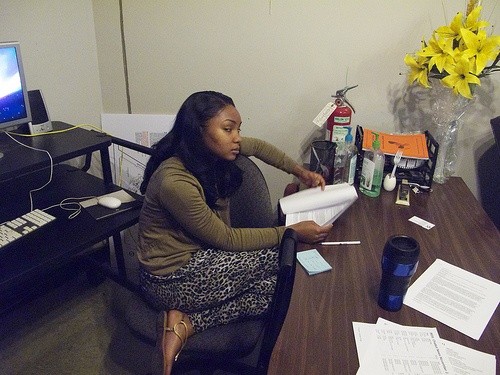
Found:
[0,41,32,151]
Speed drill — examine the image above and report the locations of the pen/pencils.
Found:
[320,241,360,245]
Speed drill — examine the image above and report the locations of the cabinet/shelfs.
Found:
[0,121,144,292]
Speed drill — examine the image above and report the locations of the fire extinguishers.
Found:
[325,84,358,143]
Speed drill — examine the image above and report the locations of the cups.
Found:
[376,234,421,313]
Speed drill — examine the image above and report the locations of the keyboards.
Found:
[0,208,56,248]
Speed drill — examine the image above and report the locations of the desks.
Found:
[268,176,500,375]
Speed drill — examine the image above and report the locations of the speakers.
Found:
[26,88,54,136]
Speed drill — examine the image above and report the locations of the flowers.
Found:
[405,4,500,99]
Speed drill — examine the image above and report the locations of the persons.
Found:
[138,91,333,375]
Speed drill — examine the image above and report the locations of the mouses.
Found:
[99,197,122,208]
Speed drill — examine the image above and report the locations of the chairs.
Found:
[111,153,294,356]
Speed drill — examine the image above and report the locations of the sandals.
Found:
[157,309,193,375]
